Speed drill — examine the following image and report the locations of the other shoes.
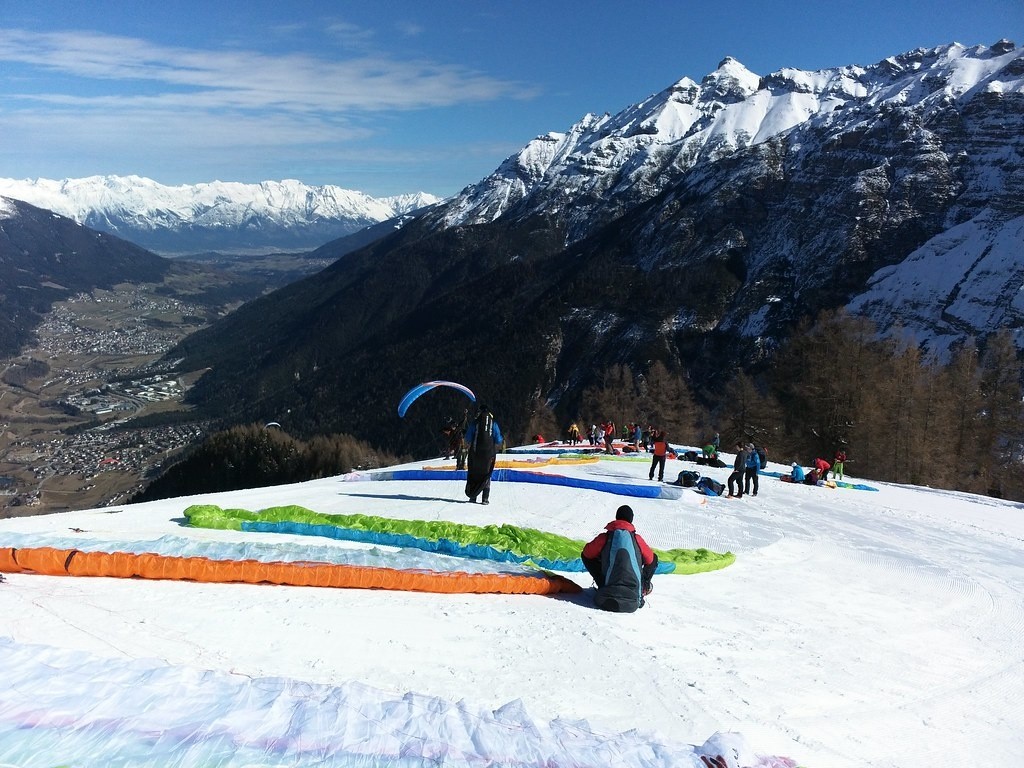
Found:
[641,581,653,598]
[469,498,477,503]
[724,495,733,498]
[481,499,490,504]
[443,457,449,460]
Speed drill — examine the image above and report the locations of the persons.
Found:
[581,505,659,595]
[649,431,670,482]
[443,404,507,505]
[703,432,720,460]
[725,442,761,498]
[562,419,678,457]
[787,451,847,483]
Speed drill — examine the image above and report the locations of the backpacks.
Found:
[593,530,646,613]
[696,476,726,496]
[611,422,616,438]
[672,470,700,487]
[755,446,767,469]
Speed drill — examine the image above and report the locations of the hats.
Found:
[607,420,612,424]
[744,443,755,448]
[792,461,797,467]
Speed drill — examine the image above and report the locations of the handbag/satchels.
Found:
[578,435,583,441]
